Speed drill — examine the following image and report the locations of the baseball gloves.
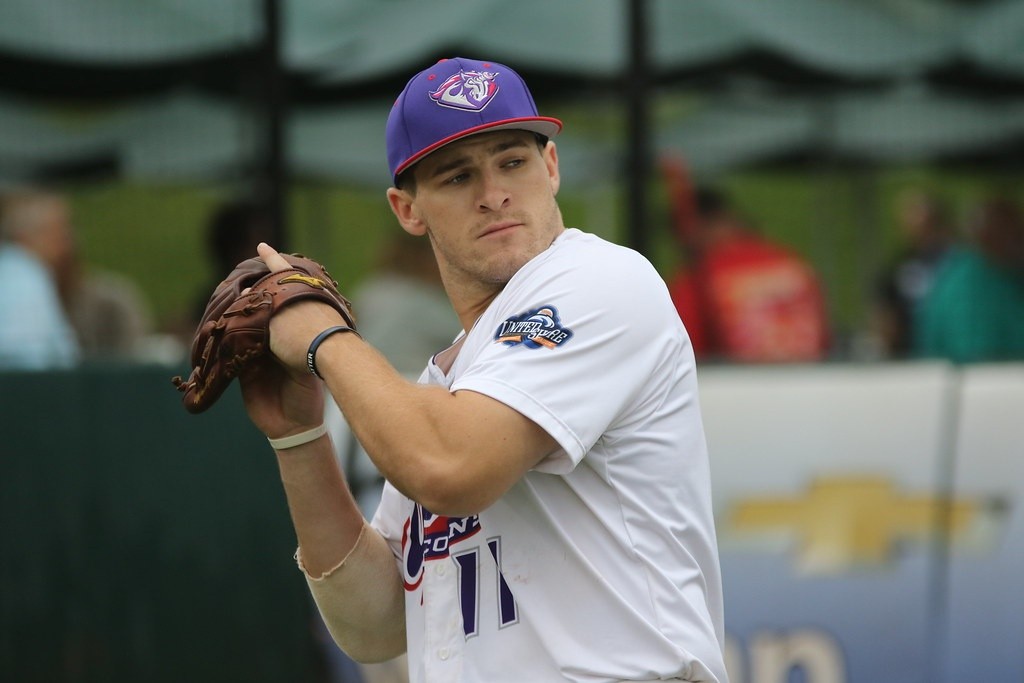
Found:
[172,249,356,415]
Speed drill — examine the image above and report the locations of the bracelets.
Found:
[267,422,328,449]
[307,325,363,381]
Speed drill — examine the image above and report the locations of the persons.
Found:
[873,187,1023,361]
[0,191,265,369]
[666,186,833,361]
[172,57,729,683]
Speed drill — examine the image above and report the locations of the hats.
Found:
[386,57,563,188]
